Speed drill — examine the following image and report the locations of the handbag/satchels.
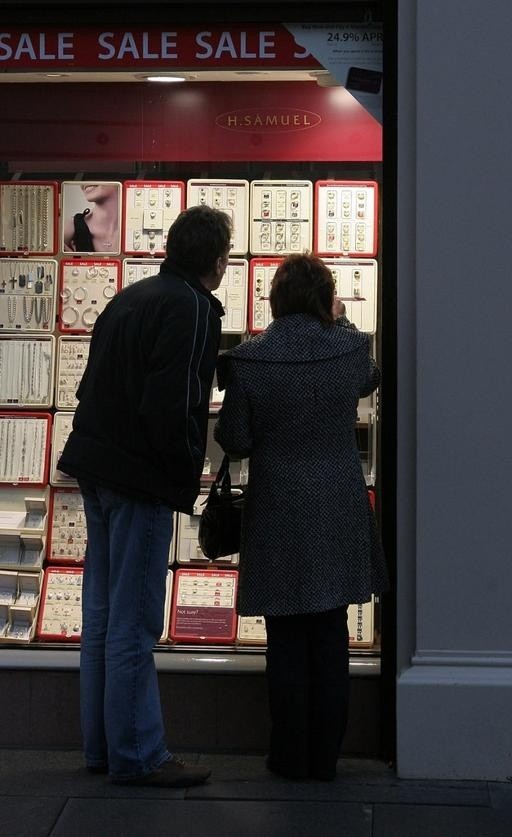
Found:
[197,456,249,559]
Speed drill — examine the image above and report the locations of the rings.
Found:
[128,183,368,256]
[174,569,269,643]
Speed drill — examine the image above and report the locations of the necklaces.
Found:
[1,183,54,481]
[103,241,113,247]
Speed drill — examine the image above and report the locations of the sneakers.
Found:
[110,753,213,790]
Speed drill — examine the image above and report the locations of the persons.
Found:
[58,205,228,787]
[196,250,386,779]
[60,182,120,255]
